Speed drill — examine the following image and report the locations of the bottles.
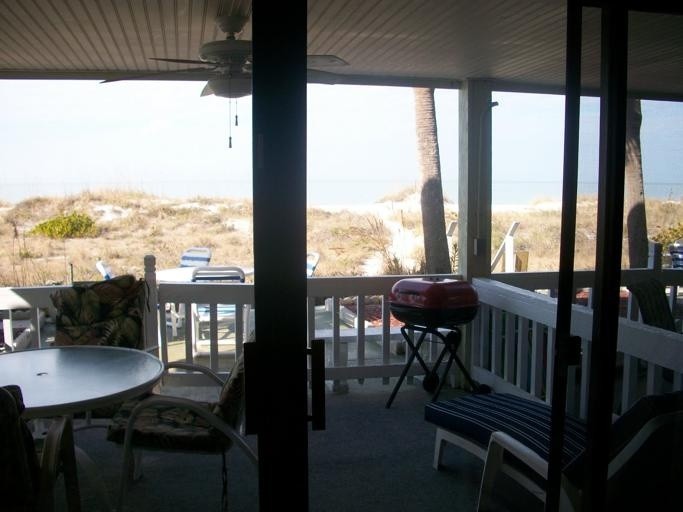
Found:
[66,262,73,286]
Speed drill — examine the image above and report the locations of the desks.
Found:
[1,343,165,512]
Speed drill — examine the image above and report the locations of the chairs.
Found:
[50,272,150,432]
[1,383,74,511]
[104,327,256,512]
[422,390,682,511]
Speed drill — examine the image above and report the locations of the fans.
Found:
[99,15,351,86]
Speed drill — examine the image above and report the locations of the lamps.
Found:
[204,71,252,100]
